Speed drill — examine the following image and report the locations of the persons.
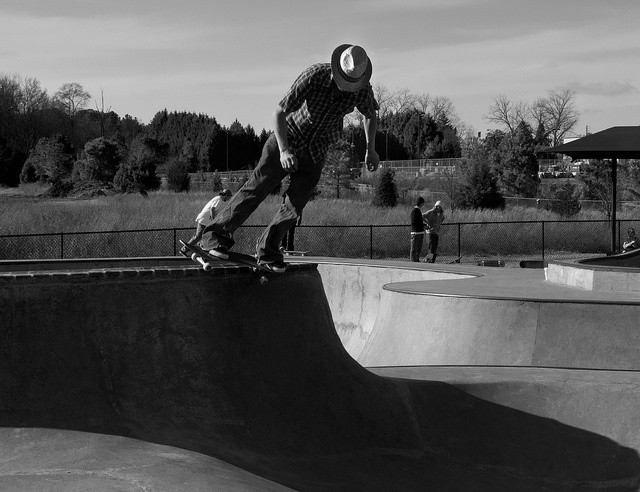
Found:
[622,228,640,252]
[281,211,302,255]
[200,44,381,272]
[410,196,424,262]
[422,200,444,263]
[180,188,232,258]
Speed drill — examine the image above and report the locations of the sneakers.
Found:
[179,250,187,258]
[262,263,285,273]
[209,247,229,259]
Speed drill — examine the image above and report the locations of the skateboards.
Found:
[178,238,274,284]
[283,249,312,256]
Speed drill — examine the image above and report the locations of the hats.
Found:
[220,189,232,196]
[331,43,372,92]
